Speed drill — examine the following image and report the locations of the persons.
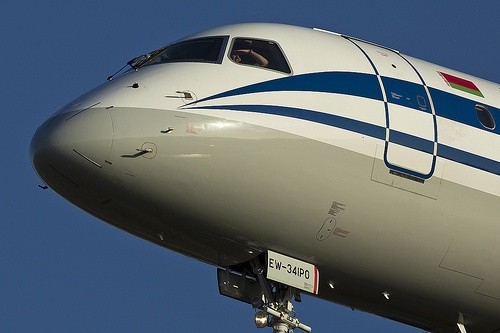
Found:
[231,48,269,67]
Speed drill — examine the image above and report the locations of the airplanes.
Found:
[29,22,499,333]
